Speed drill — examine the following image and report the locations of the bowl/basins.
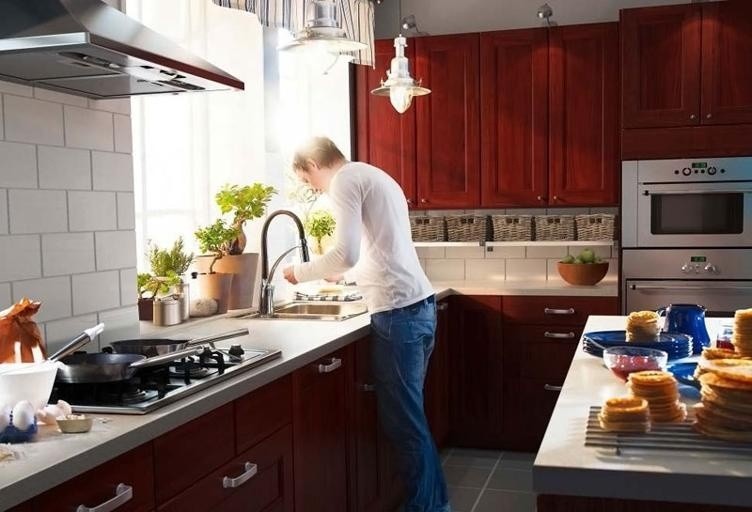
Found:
[556,262,608,286]
[603,346,668,381]
[56,414,93,432]
[1,362,58,411]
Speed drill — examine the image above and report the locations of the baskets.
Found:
[407,213,616,242]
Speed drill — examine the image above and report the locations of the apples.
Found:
[561,249,603,264]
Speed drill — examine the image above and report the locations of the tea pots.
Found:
[656,304,710,355]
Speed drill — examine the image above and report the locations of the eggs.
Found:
[0,399,34,431]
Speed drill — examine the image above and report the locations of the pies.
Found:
[627,370,688,421]
[692,346,752,380]
[598,398,651,432]
[625,310,662,342]
[730,308,752,355]
[694,358,752,441]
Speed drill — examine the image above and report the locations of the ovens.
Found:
[622,156,752,314]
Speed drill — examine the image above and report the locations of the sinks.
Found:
[276,303,359,315]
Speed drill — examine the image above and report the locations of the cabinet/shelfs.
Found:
[354,30,479,211]
[288,336,400,511]
[449,294,618,451]
[618,0,751,132]
[425,296,454,454]
[479,19,622,209]
[5,375,291,511]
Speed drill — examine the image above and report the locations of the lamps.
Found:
[274,0,370,75]
[370,0,433,114]
[402,15,429,38]
[536,3,557,28]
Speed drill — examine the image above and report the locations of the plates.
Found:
[668,360,701,389]
[583,330,693,360]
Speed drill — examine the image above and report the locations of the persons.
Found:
[283,135,450,511]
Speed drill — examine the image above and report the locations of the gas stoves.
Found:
[46,342,282,414]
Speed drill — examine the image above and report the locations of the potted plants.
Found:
[147,236,195,321]
[192,182,280,310]
[137,273,161,322]
[190,218,244,314]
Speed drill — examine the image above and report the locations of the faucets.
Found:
[259,209,311,314]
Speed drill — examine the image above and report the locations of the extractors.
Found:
[1,1,245,100]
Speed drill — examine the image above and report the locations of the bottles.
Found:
[715,324,735,350]
[152,296,185,326]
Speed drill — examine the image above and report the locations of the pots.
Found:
[110,328,250,355]
[59,346,204,383]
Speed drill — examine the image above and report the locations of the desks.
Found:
[531,312,752,511]
